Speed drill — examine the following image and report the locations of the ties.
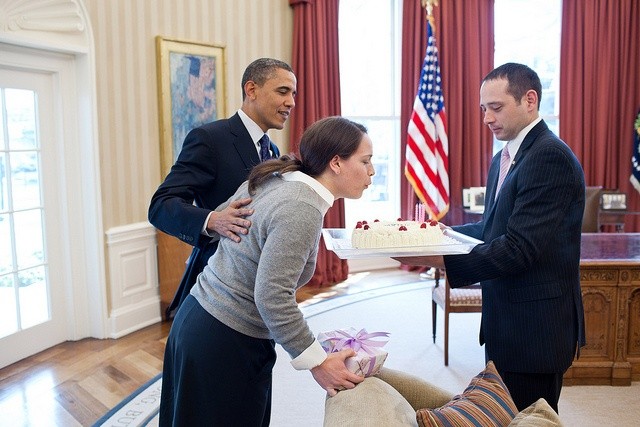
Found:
[259,134,272,161]
[495,145,510,199]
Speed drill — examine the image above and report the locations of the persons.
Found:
[158,115,375,426]
[393,62,586,416]
[146,57,298,313]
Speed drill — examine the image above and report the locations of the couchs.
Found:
[323,359,457,427]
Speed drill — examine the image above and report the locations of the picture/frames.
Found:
[156,35,228,182]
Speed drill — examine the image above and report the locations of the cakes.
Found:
[349,219,441,250]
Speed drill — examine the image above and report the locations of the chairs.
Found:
[430,265,484,366]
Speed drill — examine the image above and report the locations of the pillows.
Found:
[416,359,519,427]
[505,397,565,427]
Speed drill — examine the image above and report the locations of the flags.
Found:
[404,19,452,221]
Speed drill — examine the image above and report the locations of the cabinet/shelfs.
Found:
[559,260,639,387]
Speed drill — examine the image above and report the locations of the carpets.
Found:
[89,274,639,427]
